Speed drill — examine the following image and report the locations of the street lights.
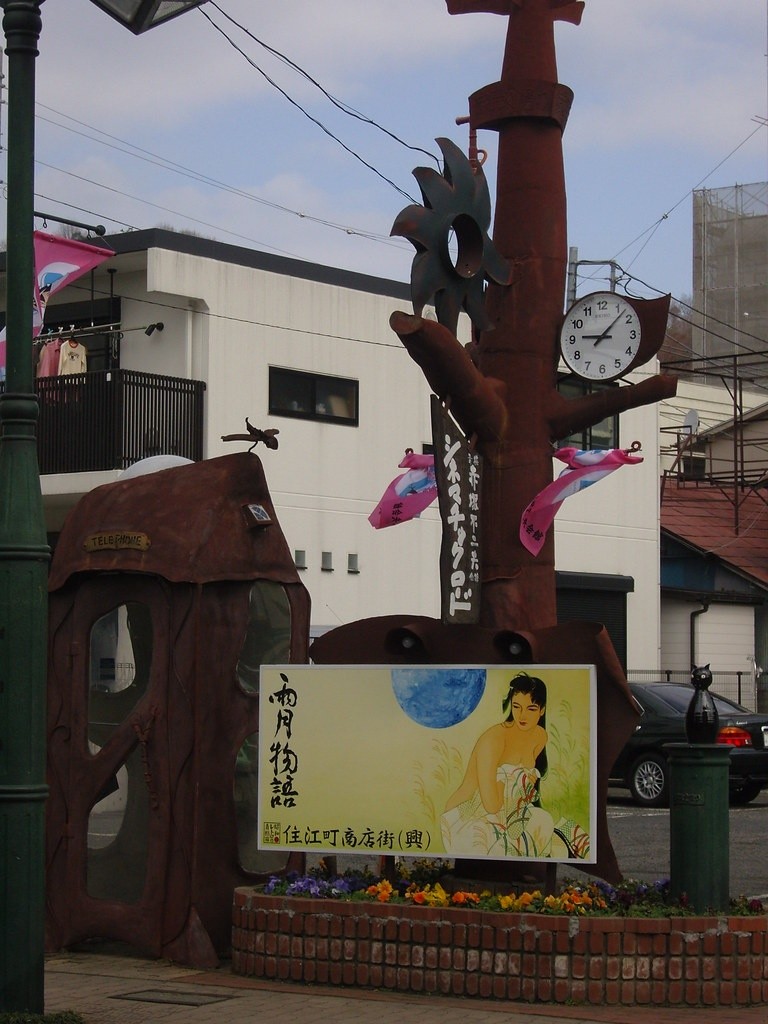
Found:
[0,0,206,1024]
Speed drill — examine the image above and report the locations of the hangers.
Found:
[34,331,85,348]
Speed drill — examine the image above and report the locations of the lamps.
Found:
[145,322,164,337]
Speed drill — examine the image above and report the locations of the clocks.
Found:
[561,292,643,381]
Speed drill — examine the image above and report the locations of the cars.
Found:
[607,681,768,808]
